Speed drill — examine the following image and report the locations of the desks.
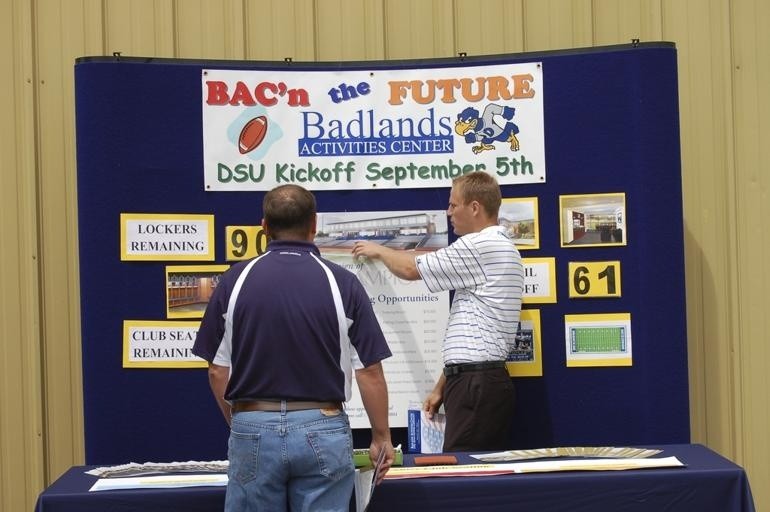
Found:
[33,444,757,512]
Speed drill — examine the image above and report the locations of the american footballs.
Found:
[239,116,267,154]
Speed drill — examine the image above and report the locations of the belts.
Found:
[442,358,508,376]
[229,396,344,414]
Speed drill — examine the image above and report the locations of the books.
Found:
[415,454,456,465]
[352,445,404,469]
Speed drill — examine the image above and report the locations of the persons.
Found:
[189,185,396,512]
[348,172,527,454]
